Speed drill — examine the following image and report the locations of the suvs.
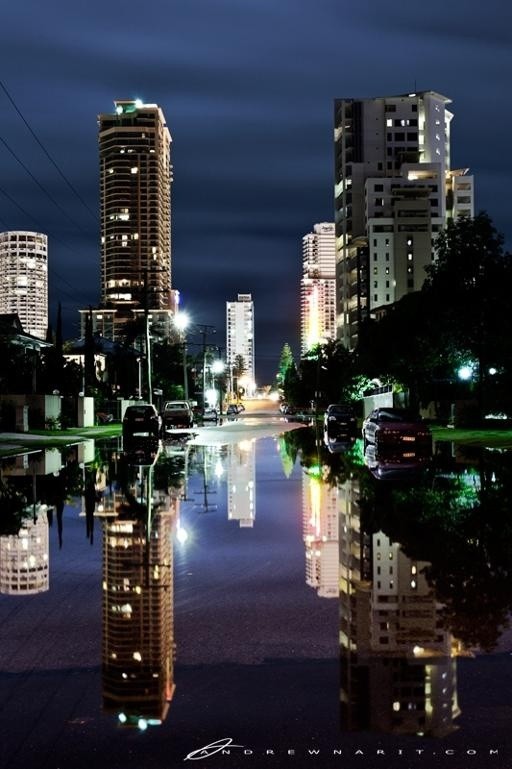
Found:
[121,403,164,438]
[163,400,194,429]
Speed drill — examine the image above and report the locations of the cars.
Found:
[227,404,245,415]
[323,404,358,438]
[279,402,296,414]
[361,407,433,458]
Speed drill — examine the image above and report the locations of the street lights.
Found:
[196,323,217,409]
